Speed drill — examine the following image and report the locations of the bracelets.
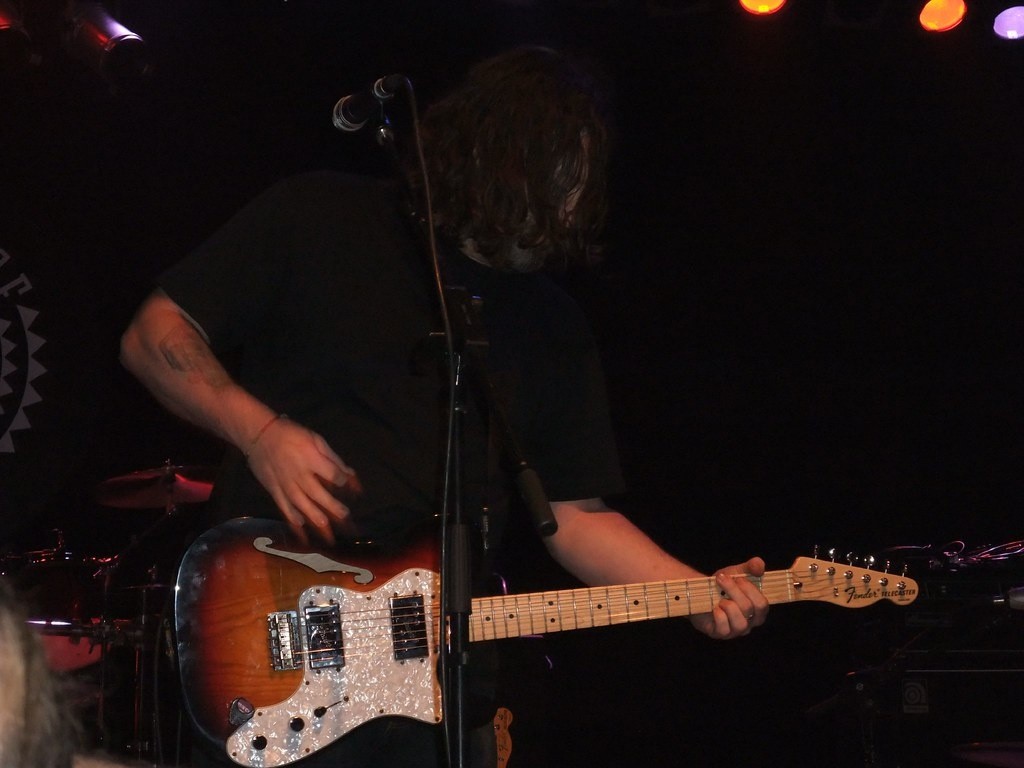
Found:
[246,416,279,460]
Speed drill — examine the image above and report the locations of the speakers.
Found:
[862,651,1024,768]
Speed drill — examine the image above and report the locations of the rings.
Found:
[747,615,755,621]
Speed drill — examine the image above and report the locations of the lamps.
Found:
[0,0,151,100]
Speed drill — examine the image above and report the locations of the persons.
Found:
[121,46,769,768]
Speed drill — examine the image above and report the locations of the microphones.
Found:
[333,73,402,132]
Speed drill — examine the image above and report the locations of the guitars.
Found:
[171,514,919,768]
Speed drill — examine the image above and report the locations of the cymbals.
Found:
[123,583,168,590]
[93,473,213,507]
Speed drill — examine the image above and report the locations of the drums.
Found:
[20,550,120,673]
